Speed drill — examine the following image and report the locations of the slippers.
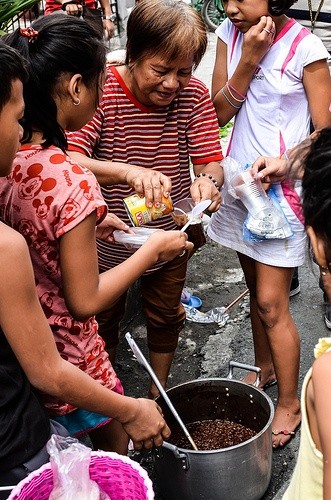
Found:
[271,405,301,449]
[180,290,202,308]
[240,371,277,392]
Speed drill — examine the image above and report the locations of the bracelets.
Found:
[194,173,220,191]
[222,80,246,109]
[62,1,77,10]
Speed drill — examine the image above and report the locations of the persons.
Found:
[211,0,331,447]
[280,128,330,500]
[65,0,224,398]
[46,0,116,41]
[0,44,170,500]
[1,14,195,456]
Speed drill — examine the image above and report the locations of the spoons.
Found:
[180,200,212,232]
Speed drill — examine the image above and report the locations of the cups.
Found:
[182,288,193,305]
[173,197,207,247]
[229,168,276,220]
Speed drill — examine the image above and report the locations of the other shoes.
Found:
[289,279,300,297]
[323,293,331,329]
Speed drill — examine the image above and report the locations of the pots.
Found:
[152,360,274,500]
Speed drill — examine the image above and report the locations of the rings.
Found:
[263,28,274,34]
[179,250,185,257]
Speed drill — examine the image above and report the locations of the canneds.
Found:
[122,185,174,228]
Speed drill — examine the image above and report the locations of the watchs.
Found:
[104,16,115,22]
